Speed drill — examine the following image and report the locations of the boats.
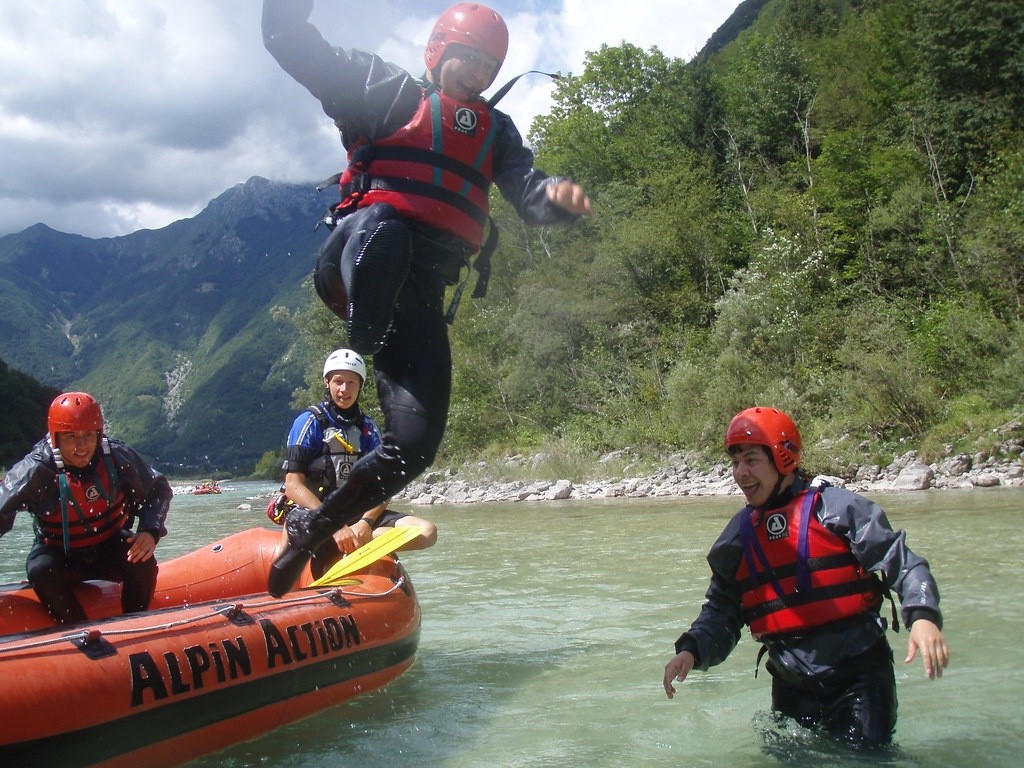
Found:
[0,527,421,766]
[192,486,223,496]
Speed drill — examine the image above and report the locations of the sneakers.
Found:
[346,218,414,356]
[266,506,343,599]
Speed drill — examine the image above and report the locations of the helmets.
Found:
[424,3,508,90]
[48,392,104,449]
[724,406,801,475]
[323,348,366,389]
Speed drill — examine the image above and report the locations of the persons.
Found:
[262,0,592,600]
[0,392,173,630]
[283,348,438,582]
[663,407,950,747]
